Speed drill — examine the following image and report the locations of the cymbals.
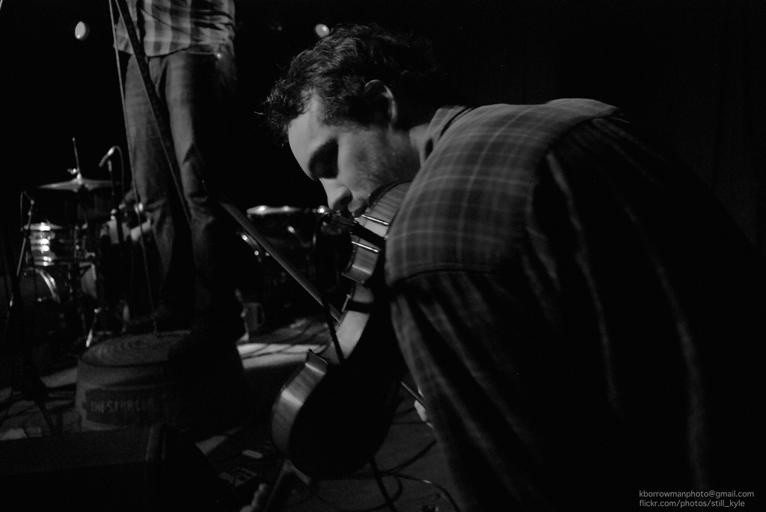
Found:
[41,179,112,191]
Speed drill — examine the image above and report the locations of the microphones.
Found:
[21,192,39,212]
[97,147,116,168]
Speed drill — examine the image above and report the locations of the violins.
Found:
[247,180,412,510]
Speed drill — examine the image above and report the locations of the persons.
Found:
[250,22,765,510]
[112,0,238,346]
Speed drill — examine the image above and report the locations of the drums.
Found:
[17,263,99,343]
[21,224,67,267]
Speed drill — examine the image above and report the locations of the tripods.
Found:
[0,217,74,438]
